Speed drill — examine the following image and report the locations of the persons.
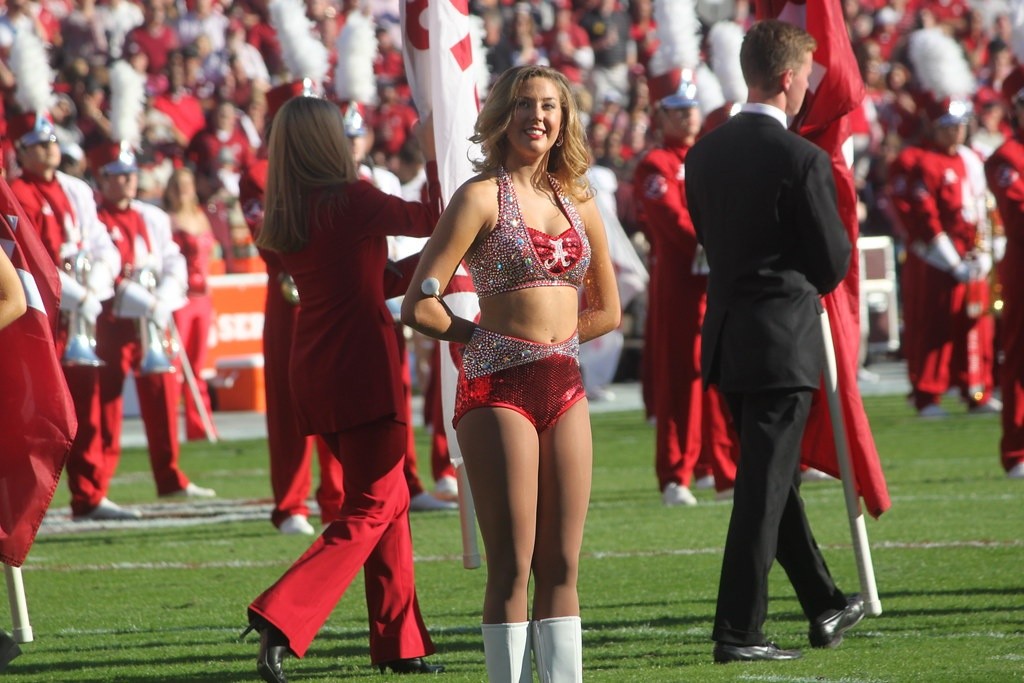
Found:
[0,0,425,273]
[9,13,141,520]
[0,248,27,332]
[632,0,738,507]
[89,59,217,499]
[850,0,1024,235]
[886,27,1003,416]
[401,65,622,683]
[984,0,1024,476]
[238,2,345,533]
[236,96,441,682]
[684,20,864,663]
[162,167,216,440]
[476,0,651,231]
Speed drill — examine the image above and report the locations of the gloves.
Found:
[927,236,994,278]
[118,273,189,331]
[49,255,121,318]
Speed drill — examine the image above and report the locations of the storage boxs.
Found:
[215,352,268,414]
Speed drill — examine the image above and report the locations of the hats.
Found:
[89,59,147,182]
[331,14,378,136]
[911,27,978,129]
[641,1,704,110]
[5,31,64,148]
[263,2,330,119]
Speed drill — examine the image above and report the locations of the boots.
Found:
[481,622,534,683]
[533,616,583,683]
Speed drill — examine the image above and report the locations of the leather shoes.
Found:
[809,597,866,649]
[714,641,803,664]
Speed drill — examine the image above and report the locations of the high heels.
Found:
[378,657,445,675]
[237,615,291,683]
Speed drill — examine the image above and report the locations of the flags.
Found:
[398,0,485,466]
[579,181,647,392]
[0,177,79,566]
[768,1,891,520]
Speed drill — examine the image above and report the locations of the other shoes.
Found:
[664,482,697,507]
[1006,462,1023,477]
[73,497,140,520]
[972,400,1001,412]
[169,481,215,499]
[280,515,314,536]
[411,491,459,512]
[923,405,947,414]
